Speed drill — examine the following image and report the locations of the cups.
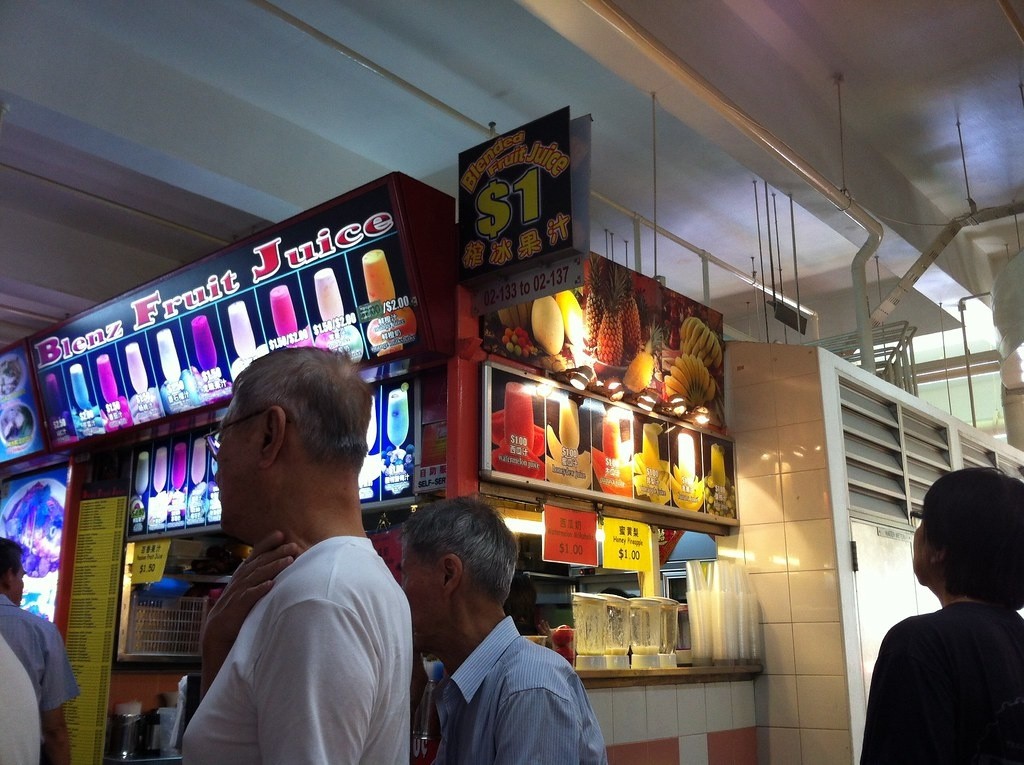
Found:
[642,423,659,468]
[362,249,395,314]
[125,342,149,394]
[558,398,580,450]
[685,558,761,665]
[96,354,119,403]
[505,381,535,450]
[314,267,344,330]
[711,444,725,487]
[549,628,576,669]
[387,388,409,450]
[602,419,617,460]
[677,434,696,481]
[191,315,217,372]
[524,636,547,648]
[228,301,256,359]
[366,396,378,454]
[270,285,297,336]
[134,438,206,498]
[156,328,182,384]
[69,363,89,410]
[45,373,63,419]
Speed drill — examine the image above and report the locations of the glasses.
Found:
[204,406,292,461]
[21,569,31,578]
[905,511,937,548]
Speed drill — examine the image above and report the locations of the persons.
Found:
[400,492,608,765]
[0,537,80,765]
[504,575,539,637]
[0,635,41,764]
[859,467,1024,765]
[182,347,413,765]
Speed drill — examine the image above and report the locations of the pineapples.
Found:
[623,316,665,391]
[623,274,641,349]
[597,262,626,366]
[584,253,604,349]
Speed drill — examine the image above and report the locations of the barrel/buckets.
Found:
[109,713,146,760]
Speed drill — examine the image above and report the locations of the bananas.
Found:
[545,425,704,511]
[666,354,715,407]
[680,316,723,370]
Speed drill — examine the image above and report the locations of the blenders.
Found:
[596,592,632,670]
[650,596,680,668]
[628,597,661,670]
[674,603,692,667]
[572,591,608,670]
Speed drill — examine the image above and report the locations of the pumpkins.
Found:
[498,302,533,329]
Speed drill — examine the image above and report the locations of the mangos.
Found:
[532,296,565,355]
[554,290,584,345]
